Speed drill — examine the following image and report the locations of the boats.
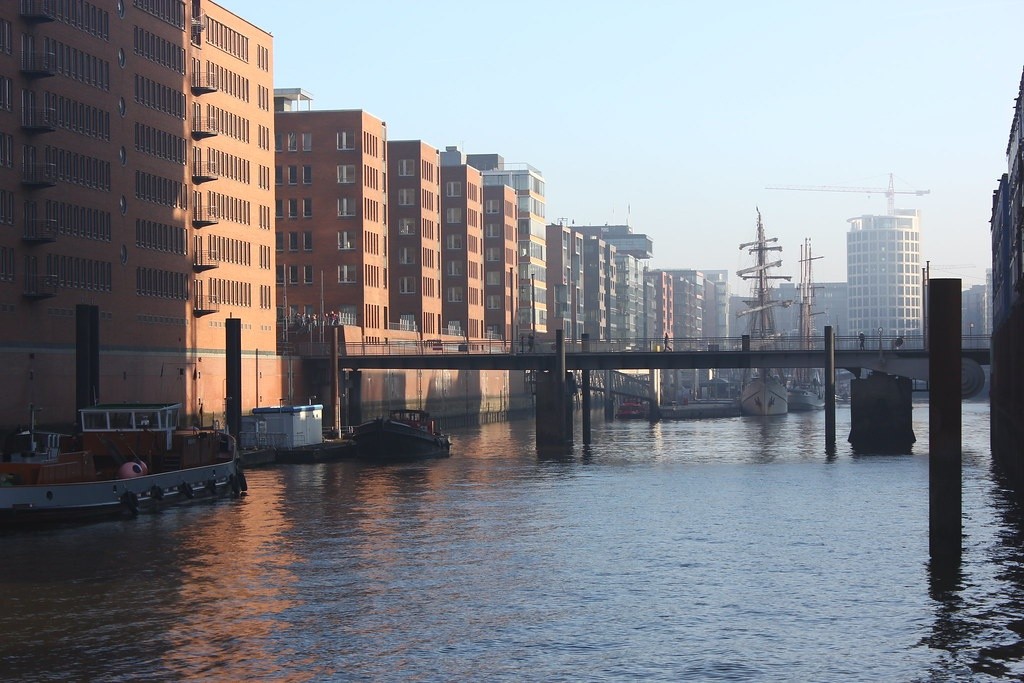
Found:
[349,409,455,461]
[0,402,250,527]
[613,205,929,422]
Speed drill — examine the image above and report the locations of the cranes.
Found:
[762,172,932,214]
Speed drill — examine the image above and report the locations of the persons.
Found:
[859,332,865,350]
[663,333,672,352]
[528,333,534,352]
[294,311,338,325]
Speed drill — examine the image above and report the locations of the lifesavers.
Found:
[126,491,141,515]
[151,485,165,500]
[205,481,216,493]
[239,472,248,491]
[231,476,240,493]
[182,482,196,499]
